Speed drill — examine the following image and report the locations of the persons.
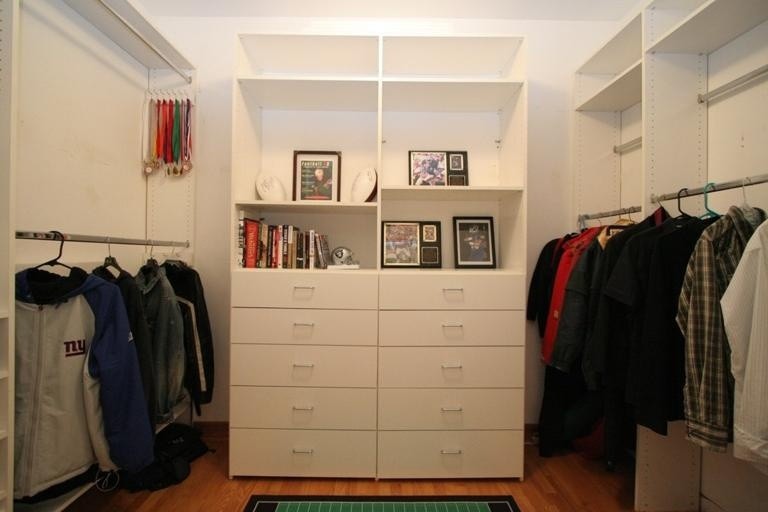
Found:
[309,167,333,196]
[415,170,434,185]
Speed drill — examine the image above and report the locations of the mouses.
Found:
[575,173,768,230]
[15,225,195,281]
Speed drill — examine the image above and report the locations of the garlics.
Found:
[144,97,193,178]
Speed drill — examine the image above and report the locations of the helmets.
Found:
[255,171,288,202]
[348,167,377,202]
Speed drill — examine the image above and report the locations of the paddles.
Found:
[241,493,521,512]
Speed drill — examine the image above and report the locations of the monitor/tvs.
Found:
[237,207,332,270]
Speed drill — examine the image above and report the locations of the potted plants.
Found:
[452,215,497,269]
[382,218,443,268]
[408,148,469,186]
[292,150,342,202]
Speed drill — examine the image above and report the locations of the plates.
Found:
[379,35,526,480]
[233,32,376,479]
